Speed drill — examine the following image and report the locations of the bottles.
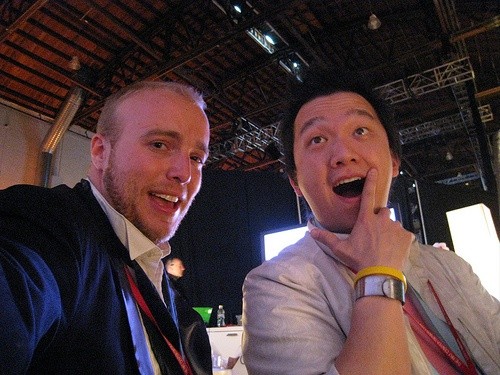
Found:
[217,305,225,327]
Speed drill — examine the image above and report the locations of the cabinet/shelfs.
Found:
[207,332,247,375]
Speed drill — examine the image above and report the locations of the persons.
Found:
[0,83,213,375]
[241,78,500,375]
[165,256,190,300]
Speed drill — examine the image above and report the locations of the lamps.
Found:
[40,87,82,154]
[67,51,81,70]
[367,5,381,30]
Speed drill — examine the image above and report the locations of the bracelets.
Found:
[353,266,408,304]
